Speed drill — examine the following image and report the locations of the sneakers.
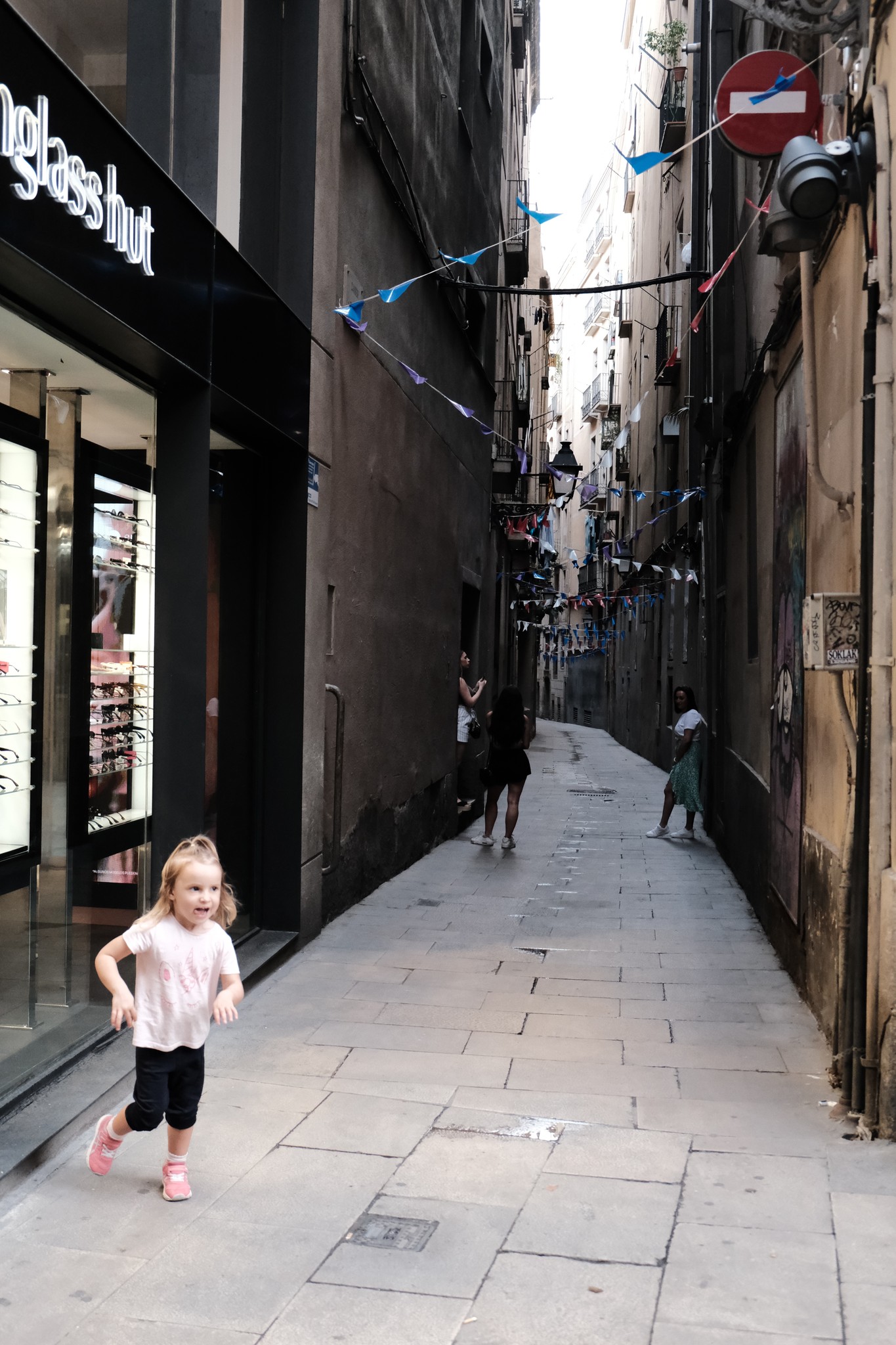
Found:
[86,1114,124,1176]
[470,830,494,845]
[501,834,516,848]
[161,1155,192,1201]
[645,822,670,838]
[670,827,696,838]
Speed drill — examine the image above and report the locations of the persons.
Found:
[90,836,244,1196]
[457,650,487,807]
[645,685,709,839]
[471,685,531,847]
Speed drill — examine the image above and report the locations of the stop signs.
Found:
[714,48,828,159]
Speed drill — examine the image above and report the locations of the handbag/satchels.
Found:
[467,710,481,739]
[479,764,494,787]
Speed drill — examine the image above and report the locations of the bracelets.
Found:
[674,757,678,763]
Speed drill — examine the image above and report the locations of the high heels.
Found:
[457,800,471,811]
